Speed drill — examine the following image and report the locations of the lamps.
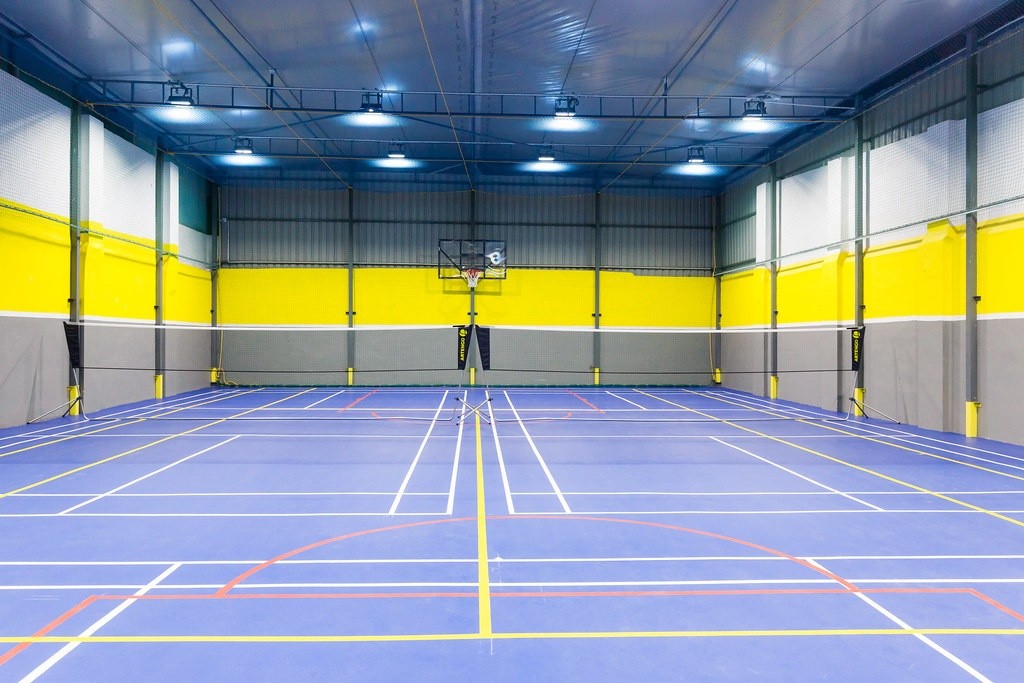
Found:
[167,87,194,105]
[687,147,705,163]
[233,138,253,153]
[360,93,383,115]
[742,100,768,120]
[387,143,406,158]
[554,98,579,116]
[538,146,555,161]
